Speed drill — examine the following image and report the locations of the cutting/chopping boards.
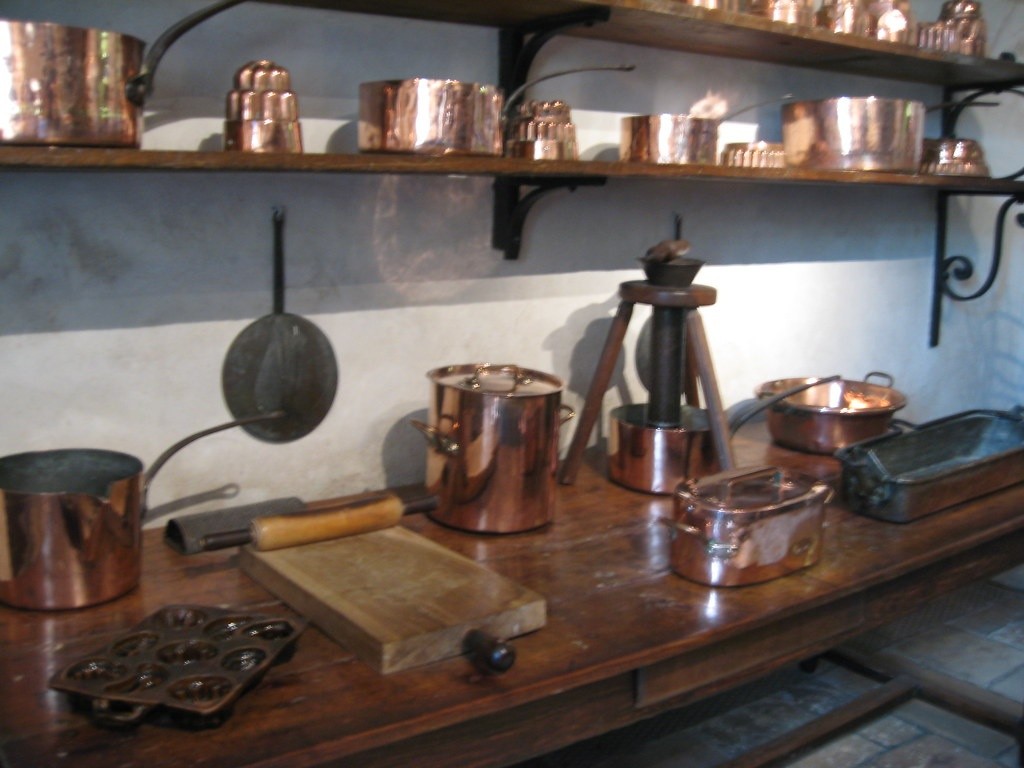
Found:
[239,524,548,678]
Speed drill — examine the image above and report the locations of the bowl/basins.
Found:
[780,96,1000,173]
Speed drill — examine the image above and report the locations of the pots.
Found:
[411,363,574,533]
[0,412,285,611]
[222,201,339,444]
[0,0,247,146]
[757,370,907,454]
[617,94,794,164]
[357,62,635,154]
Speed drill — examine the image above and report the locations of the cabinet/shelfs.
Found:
[0,0,1024,347]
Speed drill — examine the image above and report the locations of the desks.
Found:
[0,411,1024,768]
